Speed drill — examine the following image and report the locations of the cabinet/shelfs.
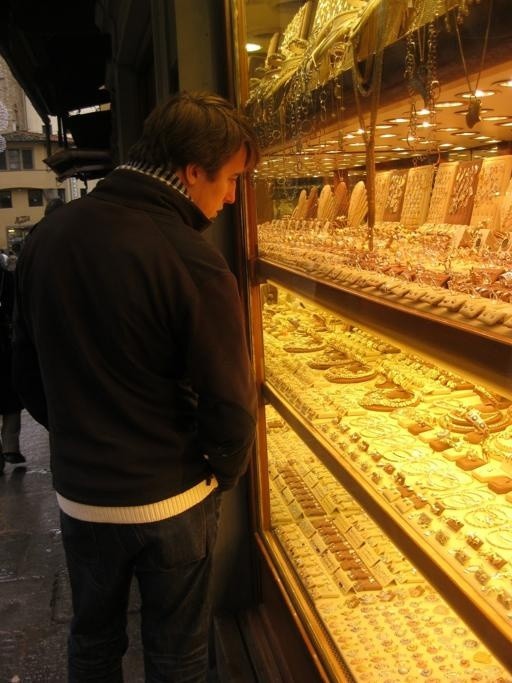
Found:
[225,0,512,683]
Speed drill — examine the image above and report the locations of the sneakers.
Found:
[6,453,25,463]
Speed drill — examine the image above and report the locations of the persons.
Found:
[12,89,260,682]
[0,243,25,472]
